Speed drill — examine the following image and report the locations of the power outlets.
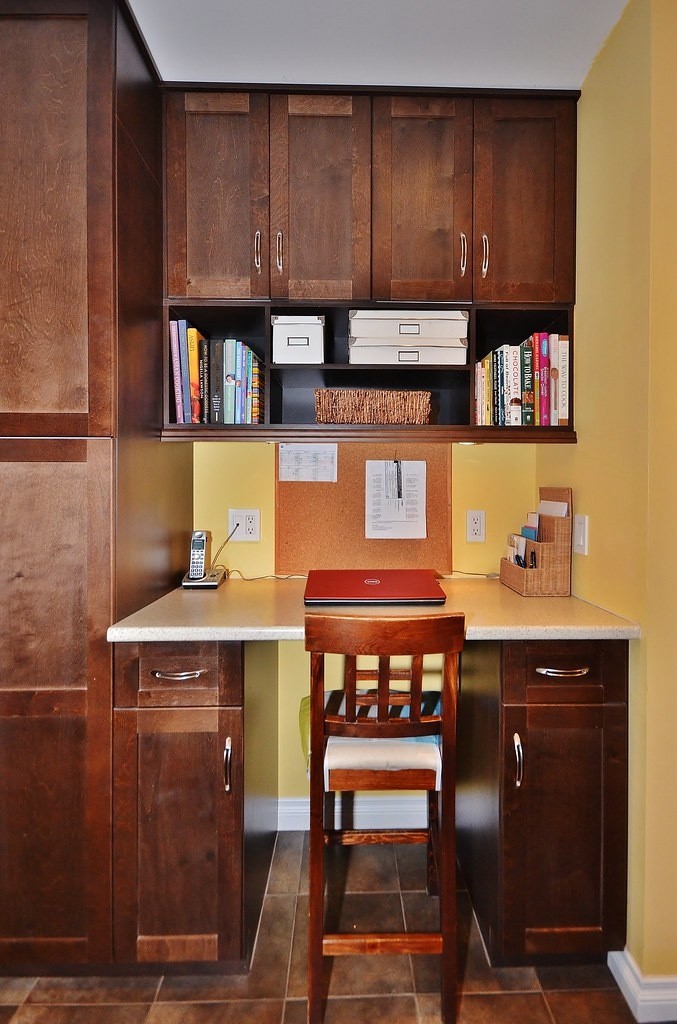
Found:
[466,510,486,544]
[227,507,261,542]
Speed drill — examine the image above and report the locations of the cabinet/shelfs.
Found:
[156,82,581,445]
[0,0,193,978]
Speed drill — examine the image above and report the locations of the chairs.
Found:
[299,609,466,1024]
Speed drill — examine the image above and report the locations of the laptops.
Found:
[302,569,447,606]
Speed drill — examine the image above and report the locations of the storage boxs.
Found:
[270,310,470,426]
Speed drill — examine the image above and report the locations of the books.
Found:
[170,320,265,424]
[475,333,569,425]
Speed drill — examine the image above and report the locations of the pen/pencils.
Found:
[515,555,526,568]
[530,547,536,568]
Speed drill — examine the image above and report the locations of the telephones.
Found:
[183,529,230,590]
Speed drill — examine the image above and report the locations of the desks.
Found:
[105,578,642,977]
